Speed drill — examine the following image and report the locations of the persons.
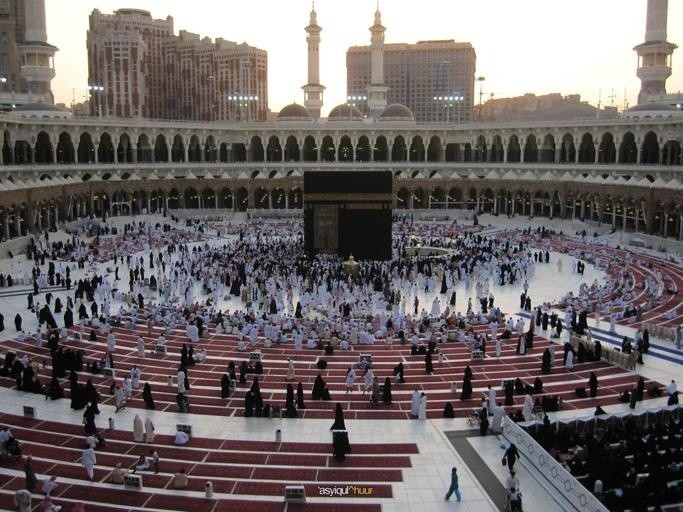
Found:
[0,204,682,512]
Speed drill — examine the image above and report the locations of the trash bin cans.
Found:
[205,481,213,497]
[276,430,282,442]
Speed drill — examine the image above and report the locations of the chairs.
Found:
[560,432,683,512]
